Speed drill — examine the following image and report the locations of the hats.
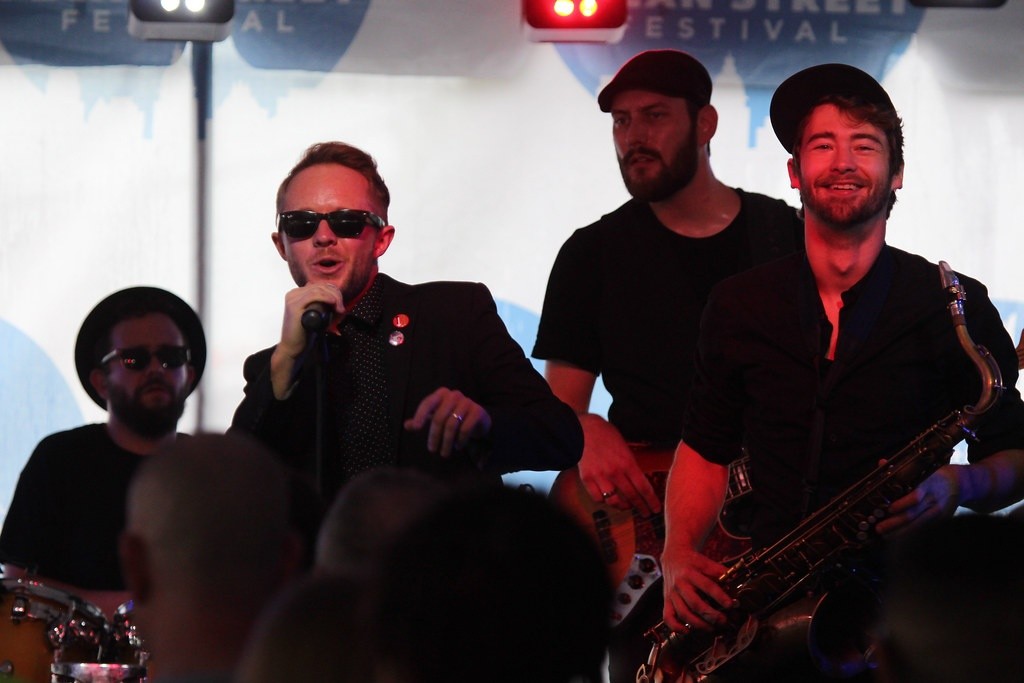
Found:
[597,48,711,113]
[76,286,207,410]
[771,61,897,151]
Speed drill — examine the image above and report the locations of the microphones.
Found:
[300,302,331,329]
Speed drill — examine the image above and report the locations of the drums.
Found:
[1,582,146,683]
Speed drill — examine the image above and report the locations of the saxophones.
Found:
[637,261,1003,683]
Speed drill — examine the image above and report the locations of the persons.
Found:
[314,465,450,588]
[659,62,1024,683]
[881,512,1024,683]
[374,484,616,683]
[117,432,304,683]
[233,577,387,683]
[225,143,584,581]
[532,49,803,683]
[1,286,206,623]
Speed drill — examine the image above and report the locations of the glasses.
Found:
[100,343,190,372]
[275,208,384,240]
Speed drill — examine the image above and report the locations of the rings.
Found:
[603,489,615,498]
[453,413,463,423]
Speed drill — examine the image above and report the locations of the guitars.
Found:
[550,443,756,627]
